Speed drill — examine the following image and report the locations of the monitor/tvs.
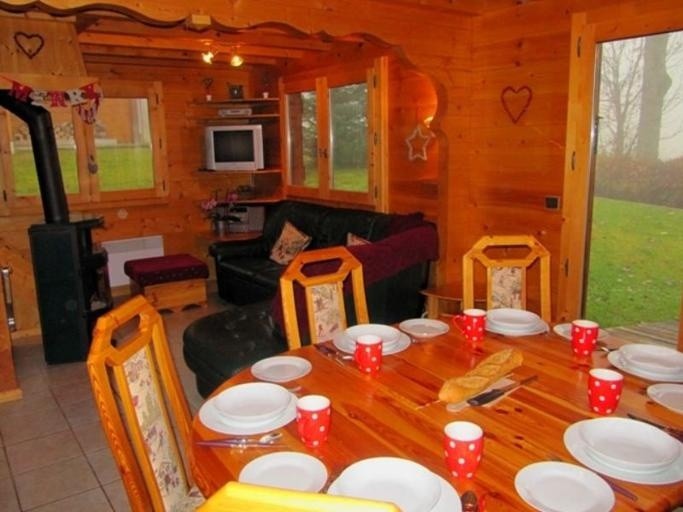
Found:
[205,124,264,171]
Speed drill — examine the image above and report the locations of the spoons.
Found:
[460,490,481,512]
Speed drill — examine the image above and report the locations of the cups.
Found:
[296,392,331,453]
[441,420,484,483]
[586,366,625,417]
[572,318,600,357]
[353,334,382,374]
[452,309,488,341]
[229,84,243,99]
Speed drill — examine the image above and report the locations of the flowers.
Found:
[197,186,247,223]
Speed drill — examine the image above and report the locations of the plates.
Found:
[329,317,451,358]
[238,452,329,498]
[326,453,465,512]
[562,414,683,487]
[513,461,615,511]
[606,342,683,414]
[482,308,609,344]
[197,380,302,439]
[254,355,314,384]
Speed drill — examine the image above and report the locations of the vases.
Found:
[214,216,228,232]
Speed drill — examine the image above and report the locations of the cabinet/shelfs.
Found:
[26,213,112,367]
[190,98,288,204]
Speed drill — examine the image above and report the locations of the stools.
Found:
[123,252,209,315]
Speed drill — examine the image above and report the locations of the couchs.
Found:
[180,199,440,399]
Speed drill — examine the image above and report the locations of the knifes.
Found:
[467,374,540,408]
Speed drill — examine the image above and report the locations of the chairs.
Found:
[84,292,206,511]
[277,245,370,352]
[459,233,553,323]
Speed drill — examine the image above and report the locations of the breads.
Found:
[439,346,523,400]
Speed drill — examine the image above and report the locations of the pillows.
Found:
[267,219,311,266]
[344,229,373,246]
